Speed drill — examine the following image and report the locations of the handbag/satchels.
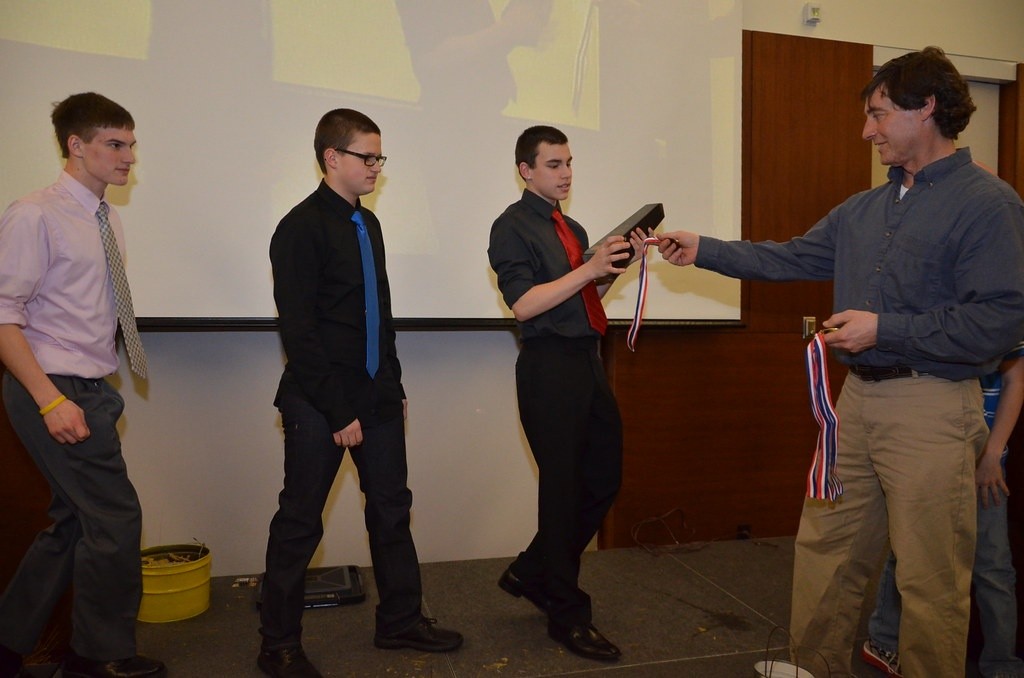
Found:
[753,626,831,678]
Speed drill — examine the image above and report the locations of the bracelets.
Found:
[39,394,66,415]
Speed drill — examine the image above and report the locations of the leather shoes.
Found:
[0,645,35,678]
[62,647,167,678]
[257,624,324,678]
[374,612,463,652]
[498,567,546,614]
[548,618,622,660]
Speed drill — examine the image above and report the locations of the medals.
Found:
[823,327,840,331]
[662,238,680,251]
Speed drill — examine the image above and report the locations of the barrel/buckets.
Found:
[754,661,815,678]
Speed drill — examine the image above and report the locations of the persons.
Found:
[648,45,1024,678]
[485,125,654,662]
[1,92,169,677]
[255,108,465,678]
[863,342,1024,677]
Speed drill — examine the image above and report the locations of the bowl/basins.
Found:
[136,543,211,622]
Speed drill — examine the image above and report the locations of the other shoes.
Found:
[861,637,902,678]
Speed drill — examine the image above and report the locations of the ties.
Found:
[551,208,608,336]
[97,202,148,379]
[350,211,380,381]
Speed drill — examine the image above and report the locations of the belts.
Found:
[847,364,930,384]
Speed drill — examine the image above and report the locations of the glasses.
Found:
[325,149,387,167]
